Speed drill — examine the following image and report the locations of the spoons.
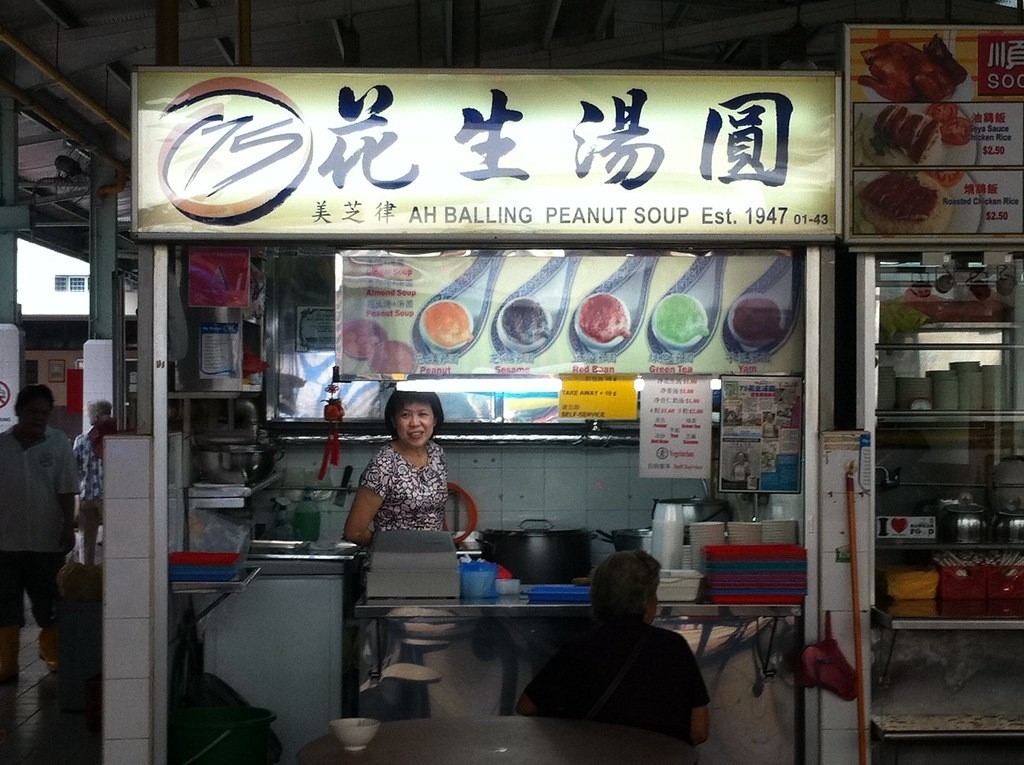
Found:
[412,256,507,362]
[723,255,799,364]
[648,256,727,364]
[569,256,660,363]
[488,255,582,361]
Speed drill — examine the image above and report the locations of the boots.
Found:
[38,626,59,673]
[0,625,20,684]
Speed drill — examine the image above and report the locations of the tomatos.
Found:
[927,103,959,124]
[927,170,964,187]
[940,116,971,145]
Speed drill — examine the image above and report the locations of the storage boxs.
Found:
[937,565,987,599]
[986,566,1024,598]
[875,564,940,599]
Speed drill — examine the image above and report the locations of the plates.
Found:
[855,103,977,168]
[682,503,797,570]
[862,66,972,102]
[854,171,981,233]
[877,362,1011,410]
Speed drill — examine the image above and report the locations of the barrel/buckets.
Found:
[173,708,278,765]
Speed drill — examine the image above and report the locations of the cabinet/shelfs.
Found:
[870,416,1024,738]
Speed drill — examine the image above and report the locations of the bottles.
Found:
[293,496,321,542]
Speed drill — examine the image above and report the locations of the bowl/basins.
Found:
[938,496,990,544]
[653,503,683,570]
[989,497,1024,544]
[342,320,386,360]
[330,718,381,750]
[370,341,418,374]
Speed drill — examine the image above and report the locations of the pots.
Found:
[184,435,285,483]
[653,499,731,545]
[475,520,598,584]
[596,526,653,556]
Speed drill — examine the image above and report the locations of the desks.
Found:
[354,597,803,765]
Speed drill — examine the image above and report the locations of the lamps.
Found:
[934,263,956,293]
[910,273,933,297]
[966,269,991,300]
[996,265,1017,296]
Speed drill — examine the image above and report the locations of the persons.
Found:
[0,384,82,684]
[731,451,751,480]
[71,401,112,564]
[344,389,449,592]
[726,407,778,436]
[515,549,710,748]
